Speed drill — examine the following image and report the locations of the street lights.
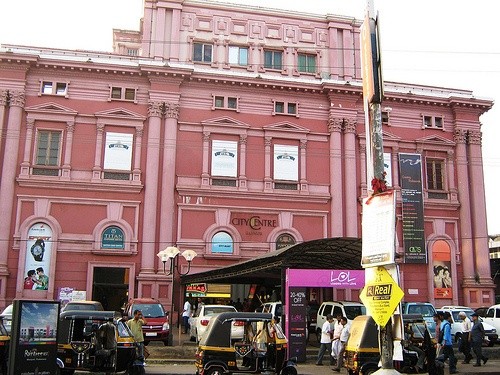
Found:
[157,241,198,345]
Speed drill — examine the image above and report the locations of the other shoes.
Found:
[332,368,340,372]
[317,364,324,365]
[484,357,489,364]
[473,363,481,367]
[461,362,470,364]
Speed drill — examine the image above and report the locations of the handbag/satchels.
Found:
[438,322,448,343]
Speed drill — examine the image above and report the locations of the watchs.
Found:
[30,239,45,261]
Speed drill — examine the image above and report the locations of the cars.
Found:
[185,305,245,338]
[1,300,28,337]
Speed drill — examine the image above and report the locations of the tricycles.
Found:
[344,315,442,375]
[197,312,297,375]
[57,312,145,375]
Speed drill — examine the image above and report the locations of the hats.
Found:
[470,312,479,317]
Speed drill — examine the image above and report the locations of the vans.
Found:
[435,306,496,347]
[316,301,367,342]
[254,301,308,345]
[125,297,173,350]
[64,299,104,310]
[404,301,436,338]
[480,302,500,341]
[445,304,471,312]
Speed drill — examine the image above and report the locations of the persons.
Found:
[24,267,49,291]
[87,309,148,365]
[180,298,285,369]
[316,311,489,374]
[433,265,452,288]
